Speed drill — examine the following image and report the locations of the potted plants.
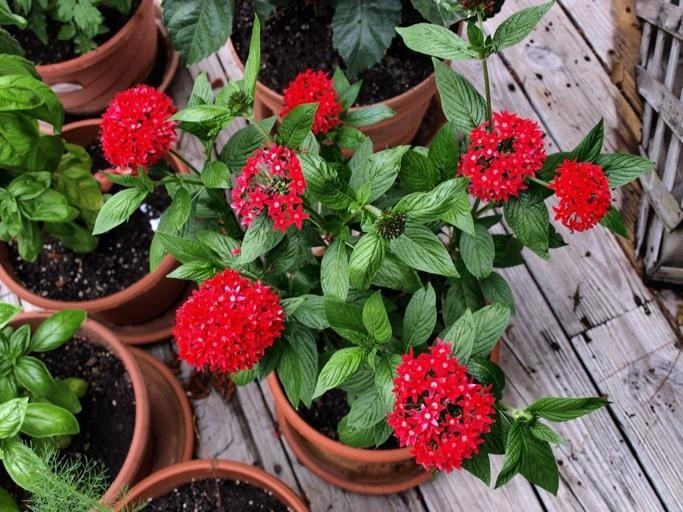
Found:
[0,305,195,512]
[0,118,200,343]
[159,0,464,156]
[0,0,181,118]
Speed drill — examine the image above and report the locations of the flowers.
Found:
[100,0,657,495]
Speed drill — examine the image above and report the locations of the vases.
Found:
[267,244,502,496]
[109,460,307,512]
